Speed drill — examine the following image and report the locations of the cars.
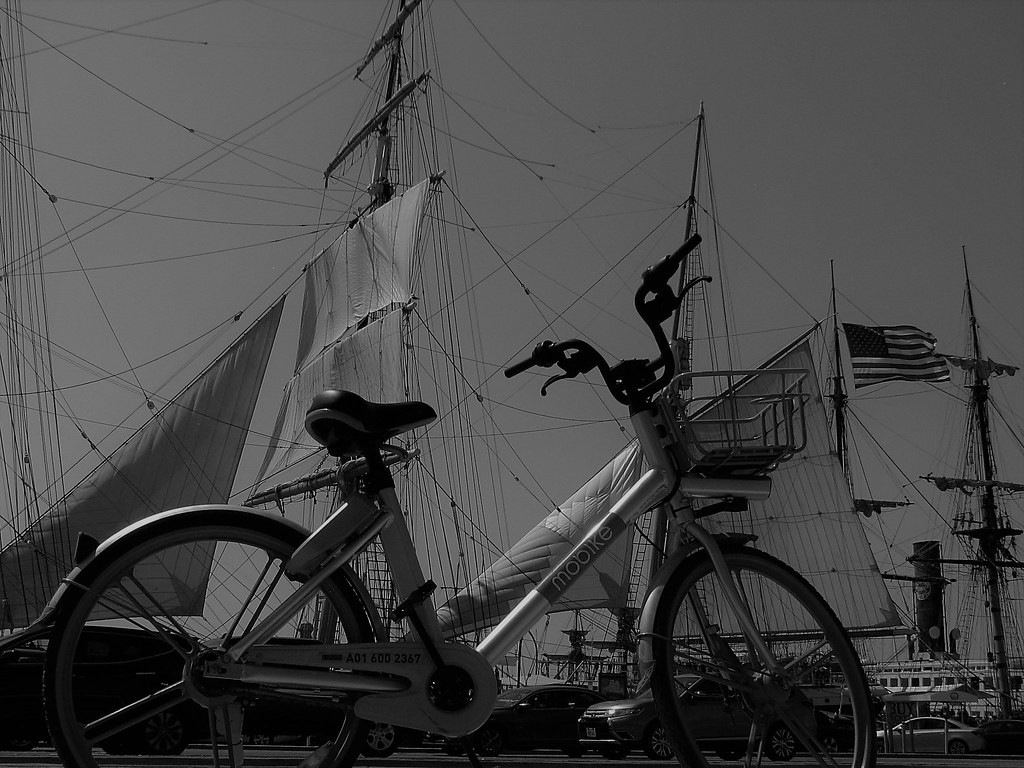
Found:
[874,713,987,755]
[970,718,1024,755]
[796,708,855,754]
[439,683,631,761]
[242,696,429,758]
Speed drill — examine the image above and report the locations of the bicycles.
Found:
[0,230,879,767]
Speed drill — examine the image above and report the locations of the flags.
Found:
[842,322,951,389]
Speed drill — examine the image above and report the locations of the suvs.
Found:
[577,674,831,763]
[0,636,226,757]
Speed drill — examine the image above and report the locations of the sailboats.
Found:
[0,0,1024,726]
